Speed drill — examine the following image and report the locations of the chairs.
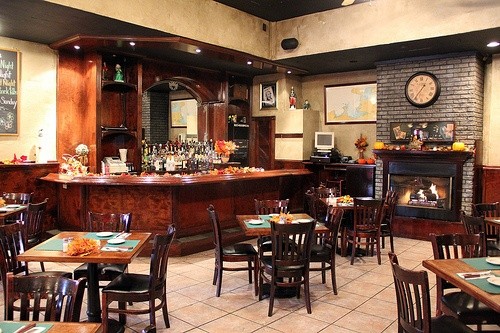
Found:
[0,184,500,333]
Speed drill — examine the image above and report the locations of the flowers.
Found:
[214,139,240,157]
[354,134,369,153]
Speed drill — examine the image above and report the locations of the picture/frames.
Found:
[323,80,378,125]
[259,79,279,111]
[390,120,456,144]
[170,97,198,128]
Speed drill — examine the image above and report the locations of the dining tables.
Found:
[422,257,500,333]
[15,232,154,333]
[318,197,389,258]
[0,203,27,225]
[235,214,328,298]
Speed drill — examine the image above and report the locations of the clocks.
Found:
[405,71,440,108]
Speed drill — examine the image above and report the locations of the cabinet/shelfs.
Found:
[301,162,376,199]
[210,72,254,168]
[57,48,141,176]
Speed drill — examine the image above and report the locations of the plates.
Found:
[485,257,500,266]
[96,232,113,237]
[297,219,311,224]
[107,239,125,244]
[6,205,20,208]
[269,213,281,217]
[487,277,500,286]
[249,220,264,225]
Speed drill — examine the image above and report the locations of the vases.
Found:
[359,152,364,159]
[221,154,228,164]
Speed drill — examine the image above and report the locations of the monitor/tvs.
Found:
[315,132,334,153]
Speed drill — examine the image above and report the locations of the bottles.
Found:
[140,139,229,174]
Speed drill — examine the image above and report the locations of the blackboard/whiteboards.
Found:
[0,48,20,136]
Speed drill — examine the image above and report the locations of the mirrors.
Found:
[141,80,207,153]
